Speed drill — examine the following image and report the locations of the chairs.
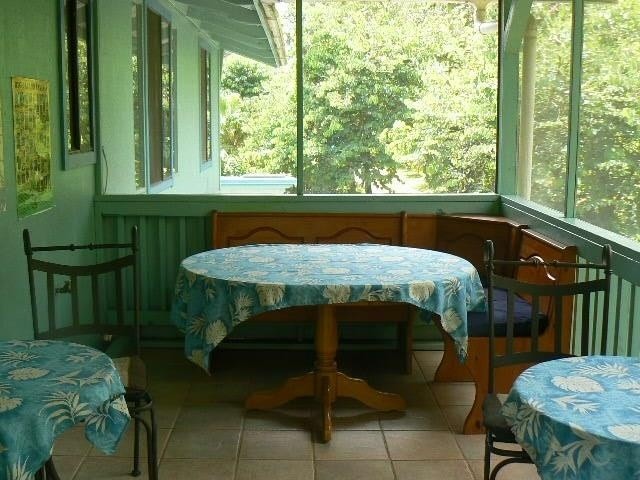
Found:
[483,240,613,480]
[23,224,159,480]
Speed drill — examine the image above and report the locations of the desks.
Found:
[503,356,640,480]
[0,337,131,480]
[174,243,486,444]
